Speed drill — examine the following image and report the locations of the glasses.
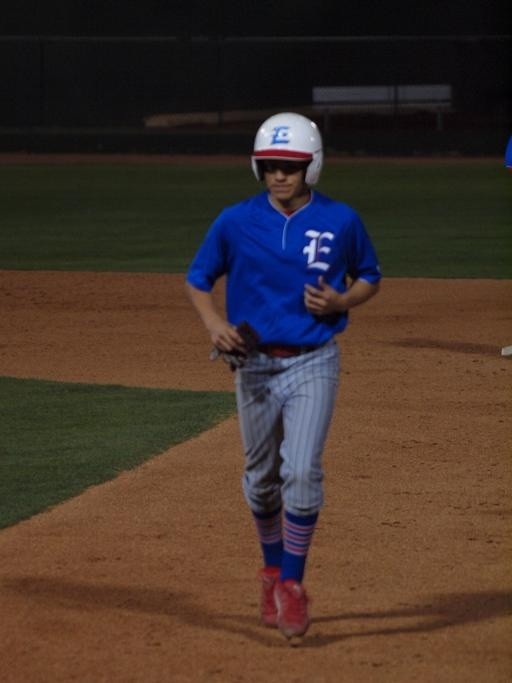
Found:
[263,165,302,176]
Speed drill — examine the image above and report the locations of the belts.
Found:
[271,344,301,358]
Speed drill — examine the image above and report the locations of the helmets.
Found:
[251,111,324,186]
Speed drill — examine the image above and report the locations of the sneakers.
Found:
[261,566,310,641]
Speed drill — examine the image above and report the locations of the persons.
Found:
[184,111,382,641]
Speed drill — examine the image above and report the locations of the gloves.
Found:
[208,319,260,373]
